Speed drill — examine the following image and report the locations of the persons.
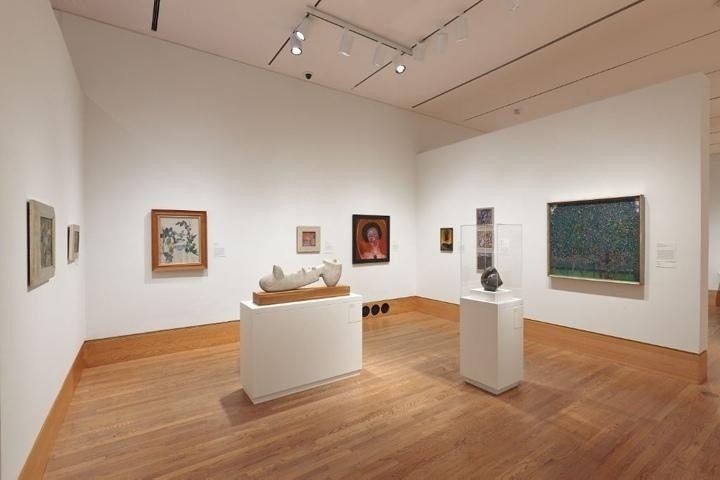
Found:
[361,222,387,260]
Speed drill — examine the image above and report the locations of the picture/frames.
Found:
[440,228,453,252]
[546,194,644,286]
[69,224,79,262]
[151,209,207,271]
[28,199,56,289]
[476,207,494,273]
[297,225,321,254]
[352,214,389,263]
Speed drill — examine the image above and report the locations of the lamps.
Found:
[353,0,519,87]
[296,6,413,75]
[289,28,302,55]
